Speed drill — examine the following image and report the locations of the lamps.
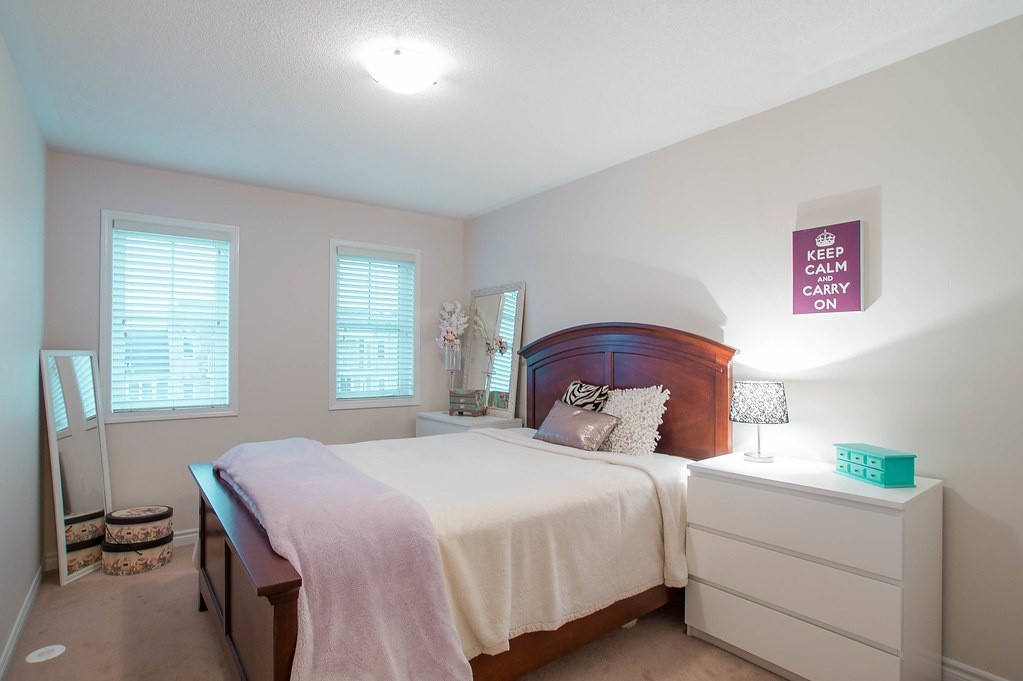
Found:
[729,379,790,463]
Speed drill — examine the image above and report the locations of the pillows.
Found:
[561,380,610,412]
[532,399,620,451]
[597,384,671,455]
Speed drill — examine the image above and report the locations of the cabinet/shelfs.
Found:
[416,411,523,438]
[685,450,942,681]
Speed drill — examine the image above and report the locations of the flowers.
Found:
[473,305,509,372]
[434,301,470,350]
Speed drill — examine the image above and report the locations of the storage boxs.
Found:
[449,389,486,416]
[101,505,175,577]
[488,390,509,409]
[832,443,917,488]
[64,508,105,575]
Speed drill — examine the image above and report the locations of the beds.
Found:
[188,320,736,681]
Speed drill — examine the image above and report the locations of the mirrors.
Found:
[462,281,526,420]
[40,349,113,587]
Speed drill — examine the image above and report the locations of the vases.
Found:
[442,346,461,414]
